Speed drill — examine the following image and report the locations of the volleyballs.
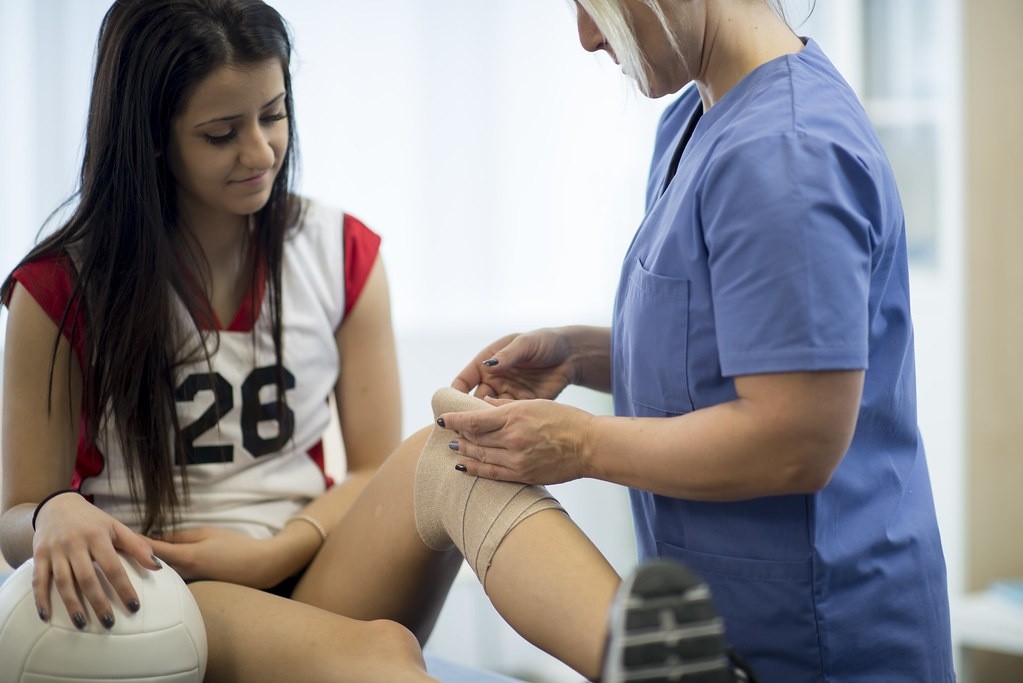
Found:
[1,554,209,683]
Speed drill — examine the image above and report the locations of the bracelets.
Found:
[288,515,327,541]
[32,491,83,530]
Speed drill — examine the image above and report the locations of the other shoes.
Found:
[605,559,731,683]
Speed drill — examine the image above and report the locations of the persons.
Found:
[1,0,734,683]
[436,1,959,683]
